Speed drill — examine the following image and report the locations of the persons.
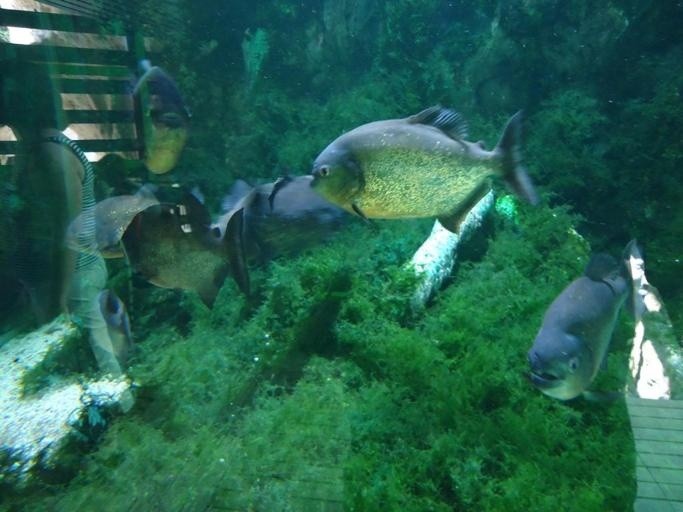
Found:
[0,60,137,415]
